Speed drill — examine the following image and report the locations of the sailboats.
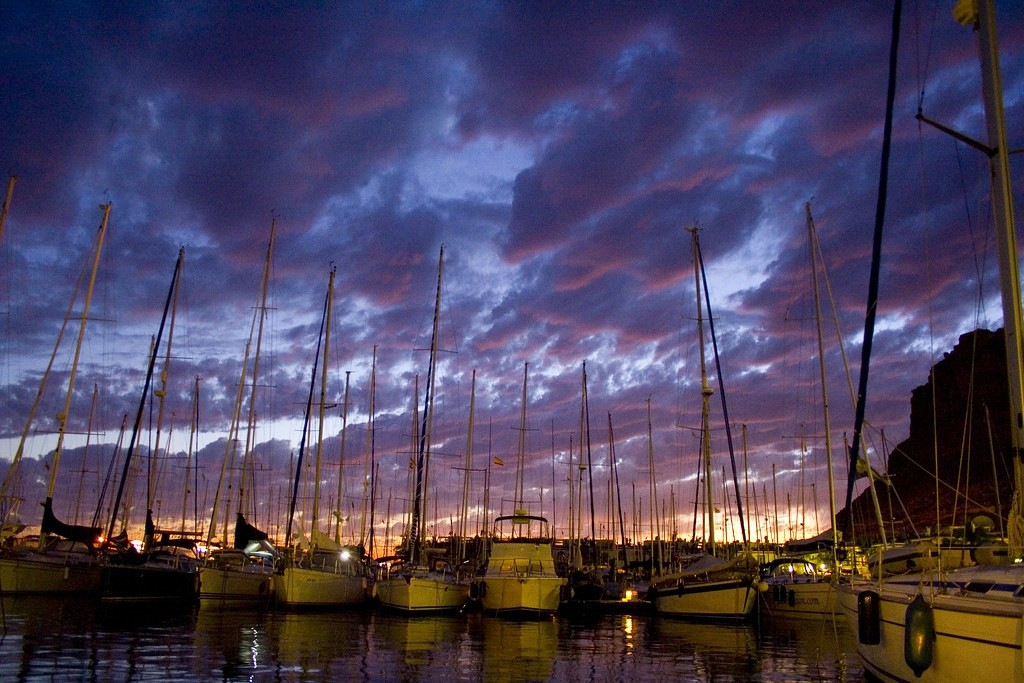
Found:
[0,0,1024,683]
